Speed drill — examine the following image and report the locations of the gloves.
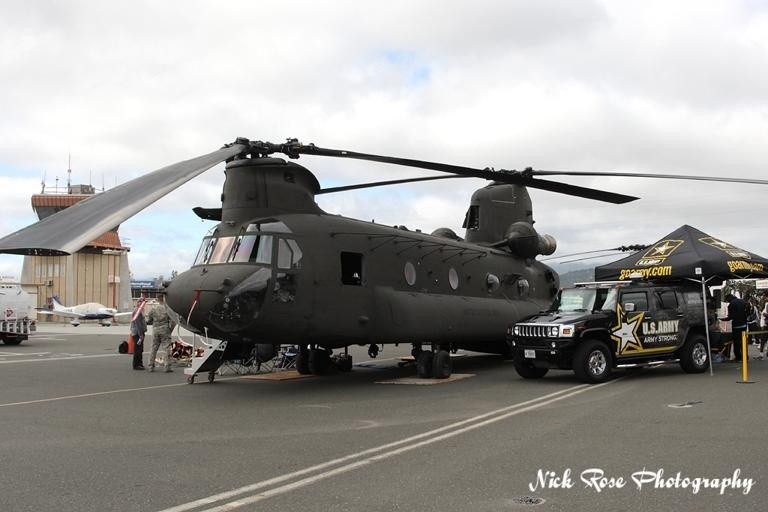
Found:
[137,339,142,345]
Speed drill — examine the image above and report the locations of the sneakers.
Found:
[164,368,174,372]
[148,366,154,372]
[753,355,764,360]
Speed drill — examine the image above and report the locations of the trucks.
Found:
[0,284,38,345]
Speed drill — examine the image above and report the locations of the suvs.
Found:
[507,280,717,383]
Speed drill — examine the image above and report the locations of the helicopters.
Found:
[0,137,768,384]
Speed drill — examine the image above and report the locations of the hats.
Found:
[137,297,148,302]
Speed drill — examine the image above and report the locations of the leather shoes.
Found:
[134,366,145,370]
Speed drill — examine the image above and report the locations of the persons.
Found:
[130,297,148,370]
[720,294,749,361]
[744,289,768,358]
[146,295,176,372]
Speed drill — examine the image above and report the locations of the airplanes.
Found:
[38,295,133,327]
